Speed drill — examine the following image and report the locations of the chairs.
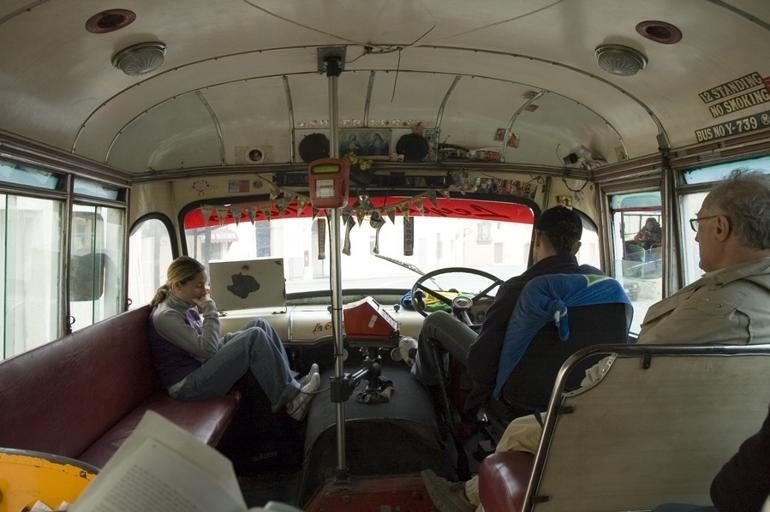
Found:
[426,302,633,482]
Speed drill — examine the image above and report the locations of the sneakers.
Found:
[397,335,419,368]
[284,371,321,425]
[296,360,324,389]
[417,465,477,512]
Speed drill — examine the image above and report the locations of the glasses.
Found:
[687,214,722,234]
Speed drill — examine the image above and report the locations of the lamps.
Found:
[112,42,169,75]
[592,45,648,77]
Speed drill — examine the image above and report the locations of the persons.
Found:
[398,204,605,391]
[634,218,662,249]
[420,169,770,511]
[149,256,321,420]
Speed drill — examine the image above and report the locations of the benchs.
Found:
[0,304,243,512]
[479,343,770,512]
